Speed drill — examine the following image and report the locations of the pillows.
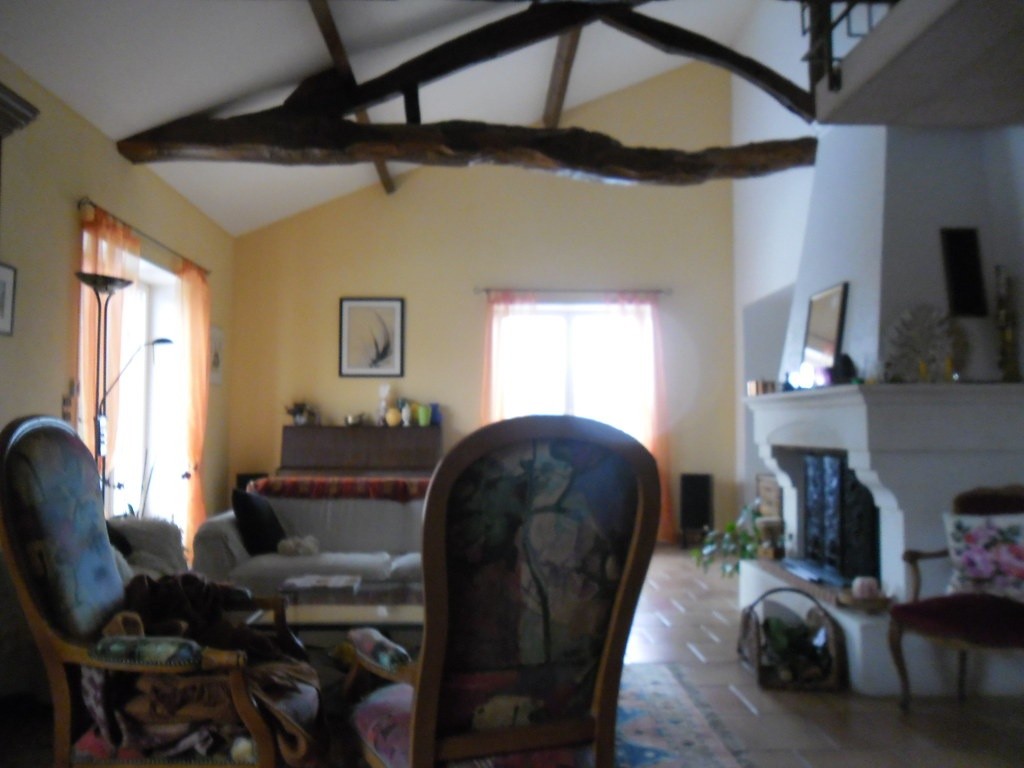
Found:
[942,511,1024,605]
[232,487,290,557]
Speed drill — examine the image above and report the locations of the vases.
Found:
[385,396,442,427]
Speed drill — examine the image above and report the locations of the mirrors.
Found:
[801,282,849,386]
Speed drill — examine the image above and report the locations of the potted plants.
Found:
[287,403,316,426]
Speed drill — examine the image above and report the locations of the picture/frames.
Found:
[0,262,17,336]
[338,297,406,378]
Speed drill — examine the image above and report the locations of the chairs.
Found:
[1,412,340,766]
[887,485,1024,714]
[328,416,660,767]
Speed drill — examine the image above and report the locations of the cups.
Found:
[416,406,432,427]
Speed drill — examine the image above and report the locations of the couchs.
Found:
[192,473,429,635]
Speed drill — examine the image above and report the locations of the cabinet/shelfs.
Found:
[280,426,443,476]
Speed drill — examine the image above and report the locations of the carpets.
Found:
[618,659,755,768]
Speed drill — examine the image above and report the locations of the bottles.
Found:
[401,404,410,427]
[385,408,400,427]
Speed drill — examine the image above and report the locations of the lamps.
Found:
[75,271,175,501]
